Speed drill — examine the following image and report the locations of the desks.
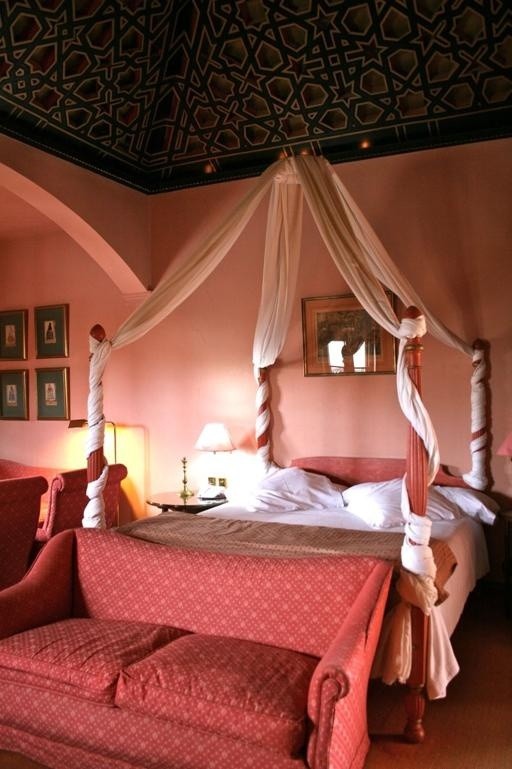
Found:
[145,492,228,513]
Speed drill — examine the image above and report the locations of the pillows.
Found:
[243,466,501,529]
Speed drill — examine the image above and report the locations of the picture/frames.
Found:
[300,290,399,376]
[0,303,72,422]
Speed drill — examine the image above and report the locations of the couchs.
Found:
[0,527,395,768]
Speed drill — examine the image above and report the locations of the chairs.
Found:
[0,464,128,590]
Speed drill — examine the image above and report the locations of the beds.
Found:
[110,457,488,744]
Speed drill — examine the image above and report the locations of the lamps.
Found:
[67,419,117,465]
[193,423,236,454]
